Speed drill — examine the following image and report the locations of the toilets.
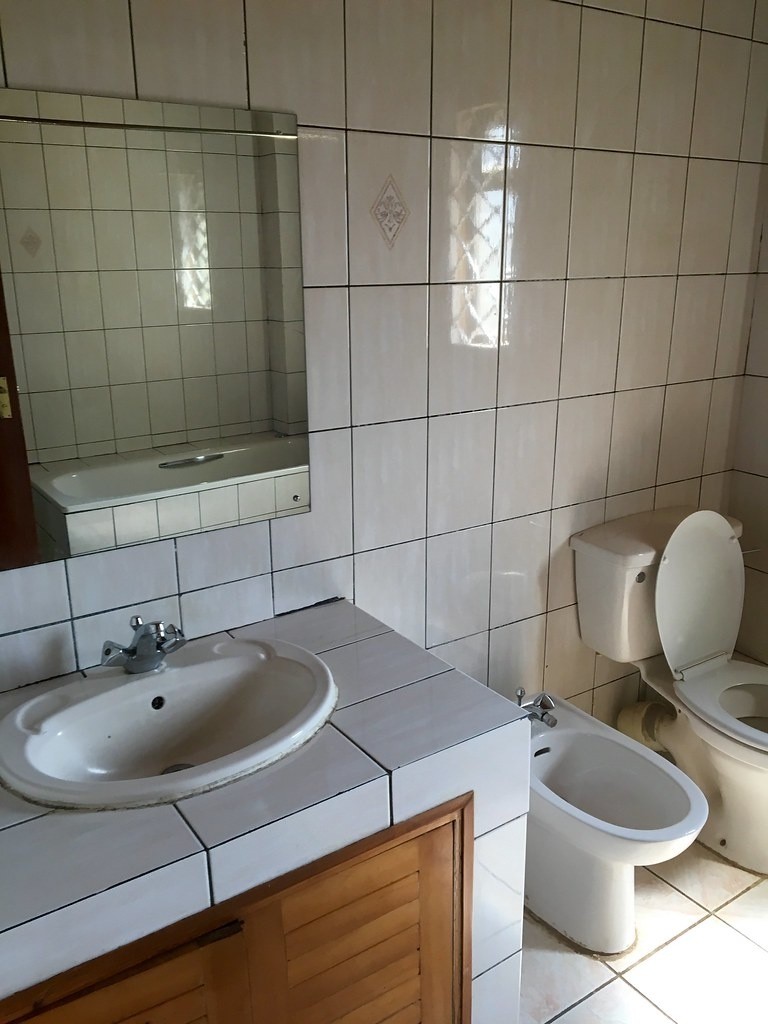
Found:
[525,690,711,955]
[566,501,768,878]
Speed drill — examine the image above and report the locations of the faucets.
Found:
[516,686,559,729]
[99,614,187,672]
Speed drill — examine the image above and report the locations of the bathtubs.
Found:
[52,435,309,509]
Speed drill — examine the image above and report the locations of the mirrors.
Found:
[0,116,299,465]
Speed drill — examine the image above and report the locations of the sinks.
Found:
[1,629,338,813]
